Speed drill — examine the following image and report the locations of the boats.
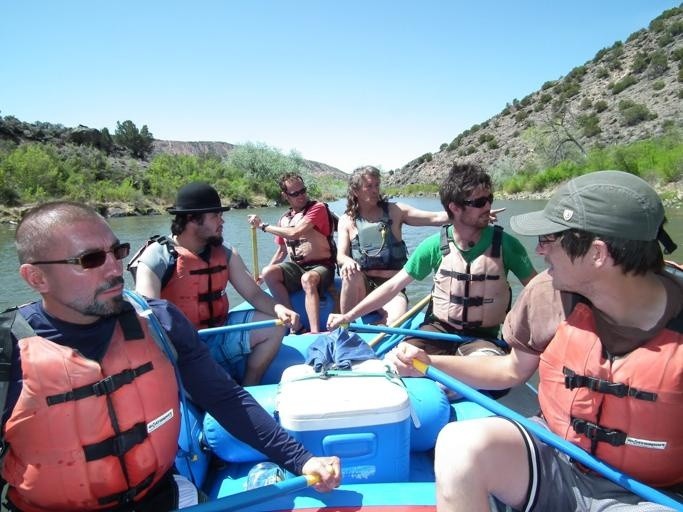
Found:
[0,275,683,512]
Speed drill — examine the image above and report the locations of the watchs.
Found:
[262,223,269,233]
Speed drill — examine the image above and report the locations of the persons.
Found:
[247,171,337,335]
[336,165,506,327]
[135,182,300,386]
[1,202,341,511]
[389,170,683,512]
[326,162,556,401]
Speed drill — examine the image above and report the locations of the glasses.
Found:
[538,235,556,248]
[463,194,492,207]
[30,243,130,268]
[285,186,306,197]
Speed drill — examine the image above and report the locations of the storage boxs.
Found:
[277,355,414,484]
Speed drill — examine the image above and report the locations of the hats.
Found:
[510,170,664,241]
[165,182,229,214]
